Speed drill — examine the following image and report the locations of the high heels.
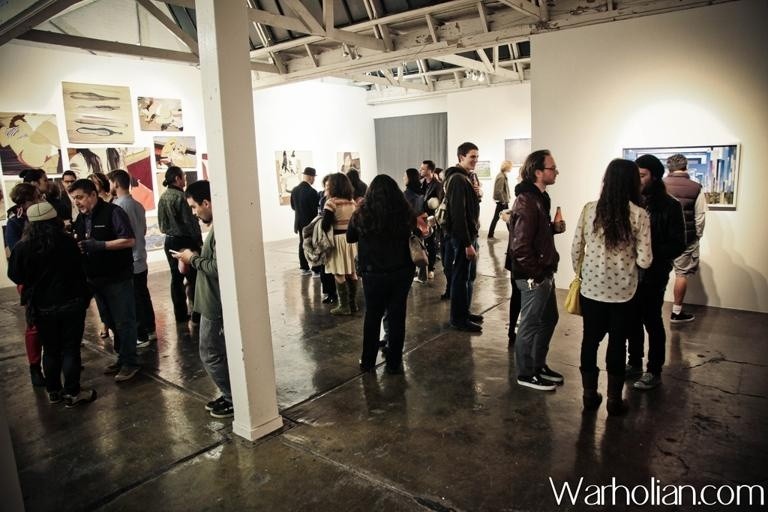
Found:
[508,332,516,338]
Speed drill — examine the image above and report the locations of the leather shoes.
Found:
[469,311,483,321]
[100,331,109,338]
[455,320,483,331]
[323,294,336,304]
[358,360,371,371]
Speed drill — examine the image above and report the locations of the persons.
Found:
[322,172,359,314]
[662,154,708,324]
[509,149,567,392]
[290,167,321,278]
[439,227,454,301]
[419,160,444,280]
[346,168,368,202]
[402,168,428,284]
[318,175,339,306]
[107,169,158,349]
[68,178,143,381]
[157,166,203,325]
[345,173,418,373]
[171,179,235,418]
[61,169,81,223]
[624,153,686,390]
[6,168,49,220]
[7,201,97,408]
[487,161,513,241]
[87,172,118,340]
[500,164,526,340]
[444,142,484,333]
[2,183,47,388]
[570,158,654,418]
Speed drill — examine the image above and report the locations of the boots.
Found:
[606,369,627,413]
[580,368,602,408]
[329,281,350,314]
[30,364,47,387]
[347,278,358,311]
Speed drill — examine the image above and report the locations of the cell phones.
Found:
[167,250,181,257]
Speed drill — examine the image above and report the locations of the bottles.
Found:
[553,206,562,234]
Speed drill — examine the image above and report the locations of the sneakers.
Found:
[633,370,662,389]
[670,310,695,322]
[487,236,499,241]
[539,363,564,384]
[210,401,234,418]
[135,339,150,348]
[147,332,157,340]
[517,374,557,390]
[413,276,424,283]
[300,268,311,275]
[311,270,320,278]
[104,362,120,374]
[63,387,96,409]
[626,362,643,376]
[115,363,141,381]
[204,396,226,410]
[48,388,63,403]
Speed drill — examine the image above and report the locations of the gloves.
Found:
[81,236,105,256]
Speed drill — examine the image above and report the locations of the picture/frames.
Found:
[202,153,210,182]
[152,134,197,169]
[156,170,198,201]
[63,83,135,145]
[138,95,184,133]
[0,111,64,176]
[335,151,360,178]
[621,142,741,210]
[66,146,156,212]
[274,149,305,206]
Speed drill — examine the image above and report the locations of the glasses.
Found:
[544,164,556,170]
[64,179,75,183]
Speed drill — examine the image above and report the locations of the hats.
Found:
[26,202,57,222]
[301,166,317,176]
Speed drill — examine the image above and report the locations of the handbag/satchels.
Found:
[408,230,429,267]
[564,202,590,316]
